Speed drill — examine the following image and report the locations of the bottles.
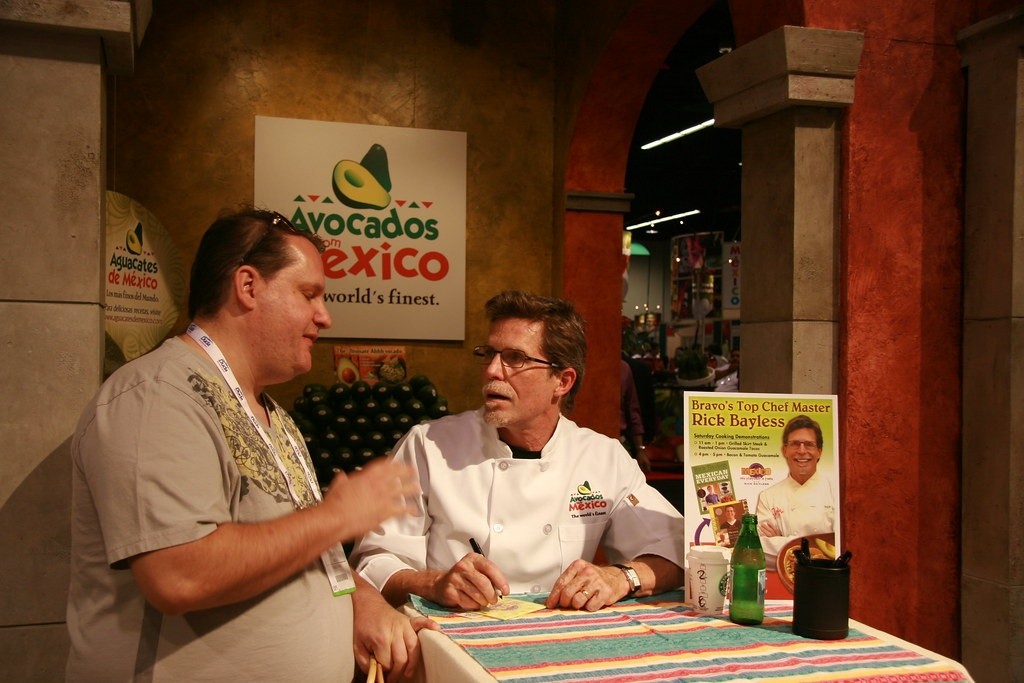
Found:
[729,514,767,625]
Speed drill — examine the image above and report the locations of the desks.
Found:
[395,586,976,683]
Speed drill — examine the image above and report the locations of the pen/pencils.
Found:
[468,537,504,601]
[793,550,809,565]
[801,538,809,556]
[835,550,852,568]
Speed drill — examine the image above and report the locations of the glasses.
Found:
[473,345,563,370]
[241,210,298,265]
[787,440,817,449]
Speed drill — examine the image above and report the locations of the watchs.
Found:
[610,564,641,601]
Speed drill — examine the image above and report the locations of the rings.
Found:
[580,590,590,600]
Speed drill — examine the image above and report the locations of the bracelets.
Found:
[637,445,646,449]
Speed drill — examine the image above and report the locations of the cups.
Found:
[686,545,731,615]
[791,560,851,640]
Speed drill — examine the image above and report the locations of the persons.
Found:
[756,415,835,568]
[706,486,720,507]
[631,342,661,359]
[720,506,742,548]
[620,360,651,474]
[715,347,740,392]
[65,203,442,683]
[347,291,685,616]
[707,343,728,367]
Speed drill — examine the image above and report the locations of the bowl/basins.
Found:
[775,532,835,595]
[371,356,406,386]
